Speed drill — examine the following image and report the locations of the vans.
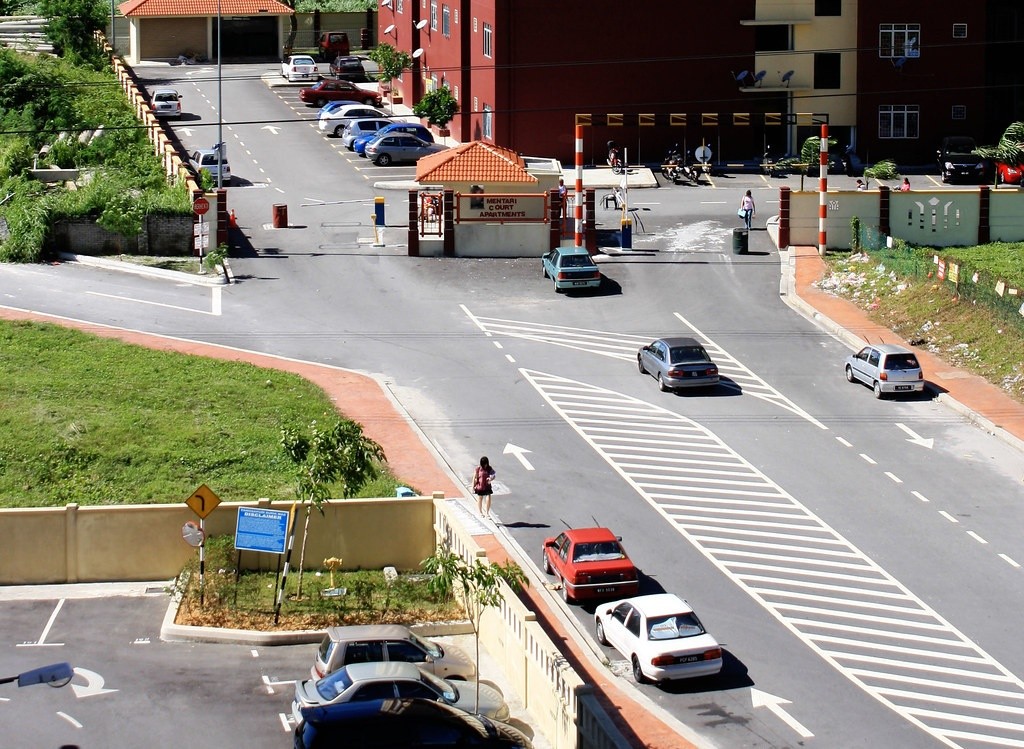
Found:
[318,31,350,59]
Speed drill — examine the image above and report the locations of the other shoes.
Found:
[480,513,484,518]
[745,225,748,229]
[749,227,751,230]
[486,514,491,520]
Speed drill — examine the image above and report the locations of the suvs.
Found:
[151,89,183,118]
[937,136,986,183]
[190,150,231,184]
[311,624,478,684]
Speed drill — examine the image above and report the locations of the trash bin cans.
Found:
[273,204,288,228]
[733,228,748,256]
[360,28,369,50]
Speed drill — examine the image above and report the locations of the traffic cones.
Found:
[230,209,235,222]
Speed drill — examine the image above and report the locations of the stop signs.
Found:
[193,197,209,215]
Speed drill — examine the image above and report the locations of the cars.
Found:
[541,247,601,293]
[637,337,720,391]
[845,343,924,399]
[541,526,640,603]
[594,592,722,683]
[294,697,532,749]
[291,661,511,725]
[989,158,1024,184]
[281,54,449,166]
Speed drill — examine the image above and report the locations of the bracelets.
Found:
[753,210,754,211]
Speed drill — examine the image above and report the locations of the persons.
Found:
[473,456,495,520]
[741,190,755,230]
[856,179,864,188]
[559,179,567,218]
[900,178,910,192]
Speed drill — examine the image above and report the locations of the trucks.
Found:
[804,136,838,175]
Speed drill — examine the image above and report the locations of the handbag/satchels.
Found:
[738,209,746,218]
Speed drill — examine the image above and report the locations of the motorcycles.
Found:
[662,144,701,184]
[763,146,775,176]
[606,140,622,175]
